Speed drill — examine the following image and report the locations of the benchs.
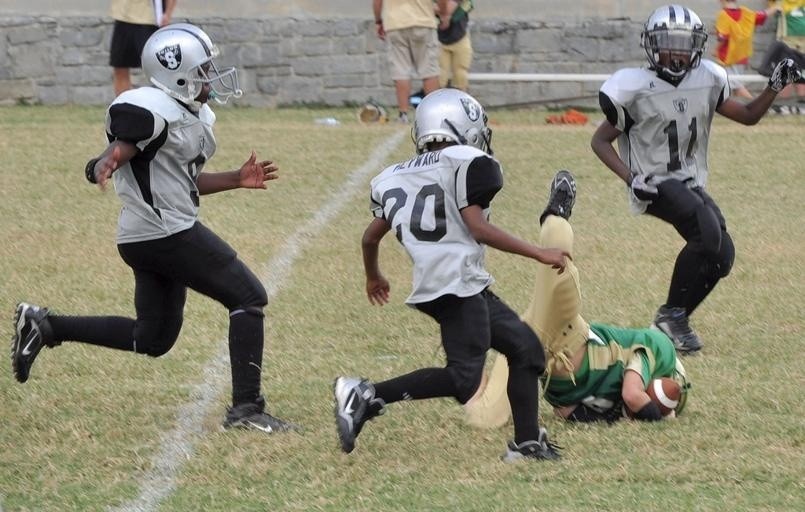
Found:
[451,69,805,114]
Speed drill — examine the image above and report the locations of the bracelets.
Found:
[374,20,383,26]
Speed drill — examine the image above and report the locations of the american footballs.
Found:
[620,378,681,420]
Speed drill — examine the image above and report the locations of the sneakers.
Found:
[538,169,577,228]
[504,425,572,467]
[12,299,60,384]
[648,304,704,352]
[332,374,388,455]
[218,395,304,439]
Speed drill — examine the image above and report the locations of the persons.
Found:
[372,0,451,127]
[764,0,805,116]
[713,0,778,116]
[589,4,805,358]
[12,20,308,441]
[445,169,690,428]
[329,85,574,471]
[100,1,180,101]
[433,0,477,97]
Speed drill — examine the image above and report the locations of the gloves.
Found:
[629,172,661,204]
[767,56,805,93]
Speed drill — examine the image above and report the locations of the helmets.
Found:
[641,4,709,84]
[412,87,490,154]
[138,23,221,104]
